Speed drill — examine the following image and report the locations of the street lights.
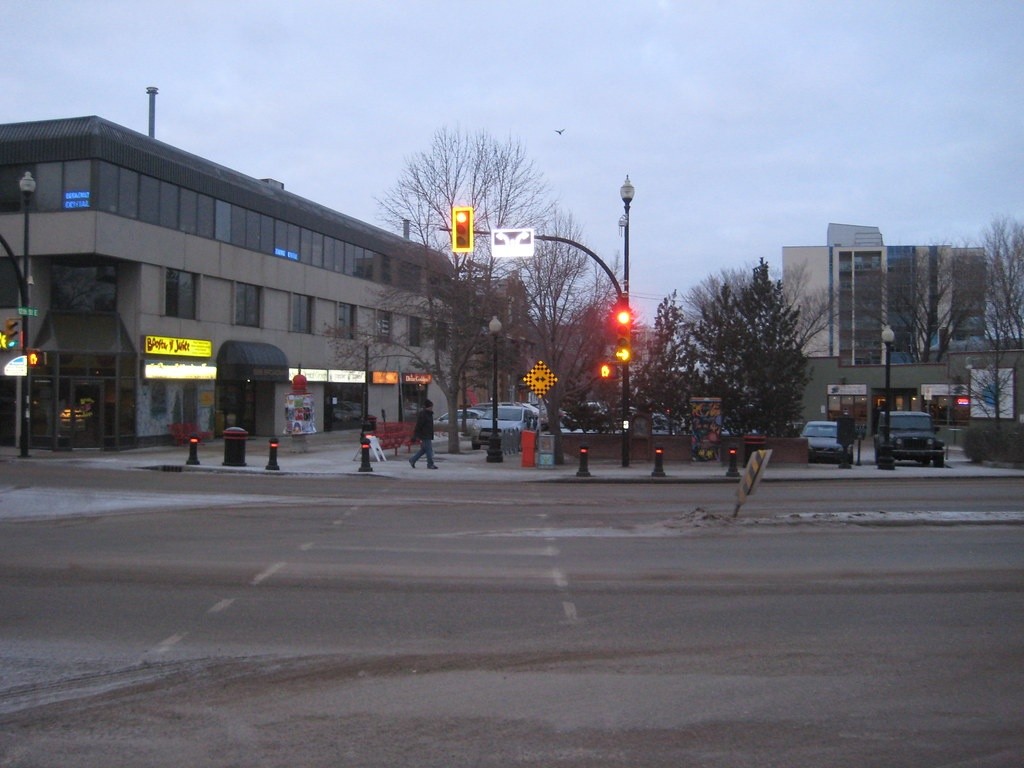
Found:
[618,176,638,468]
[362,335,374,431]
[15,170,32,459]
[486,315,506,463]
[878,325,896,469]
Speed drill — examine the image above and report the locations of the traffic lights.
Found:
[600,364,617,379]
[617,307,633,362]
[452,207,473,252]
[26,351,45,366]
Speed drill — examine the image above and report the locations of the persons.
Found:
[409,400,439,469]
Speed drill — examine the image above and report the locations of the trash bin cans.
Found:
[742,435,767,468]
[222,427,248,466]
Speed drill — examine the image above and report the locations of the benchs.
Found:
[352,422,421,462]
[168,421,214,449]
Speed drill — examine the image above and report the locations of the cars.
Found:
[432,410,486,433]
[469,401,569,429]
[472,407,537,452]
[873,412,945,469]
[799,421,854,464]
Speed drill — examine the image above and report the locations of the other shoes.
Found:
[409,459,415,468]
[427,465,438,469]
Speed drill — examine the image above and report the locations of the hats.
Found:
[423,399,433,407]
